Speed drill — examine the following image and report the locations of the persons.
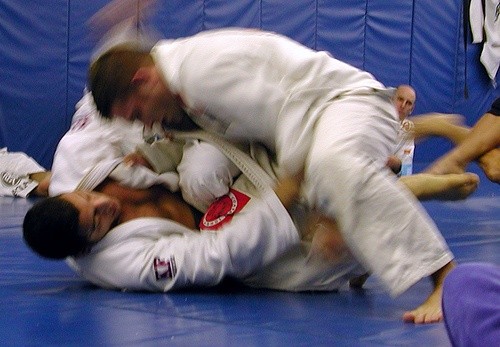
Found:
[89,27,454,325]
[1,142,52,197]
[22,86,480,292]
[391,84,500,173]
